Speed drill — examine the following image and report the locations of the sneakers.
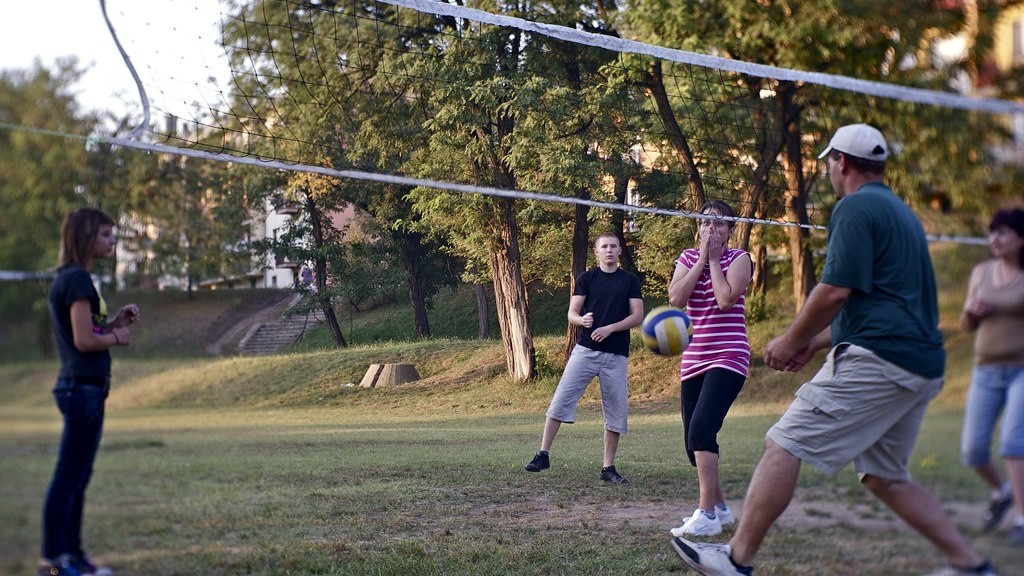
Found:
[670,536,754,576]
[926,558,999,576]
[524,451,550,472]
[982,485,1015,532]
[599,465,629,485]
[36,551,113,576]
[682,506,735,526]
[670,509,722,538]
[1009,525,1024,544]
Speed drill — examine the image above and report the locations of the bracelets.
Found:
[111,331,120,346]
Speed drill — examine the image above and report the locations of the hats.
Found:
[818,122,888,161]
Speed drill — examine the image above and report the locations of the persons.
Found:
[958,206,1023,545]
[524,232,644,486]
[302,266,314,289]
[667,200,754,539]
[668,123,999,575]
[36,207,140,576]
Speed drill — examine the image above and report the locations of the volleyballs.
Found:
[641,306,693,358]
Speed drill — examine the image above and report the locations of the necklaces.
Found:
[996,259,1015,288]
